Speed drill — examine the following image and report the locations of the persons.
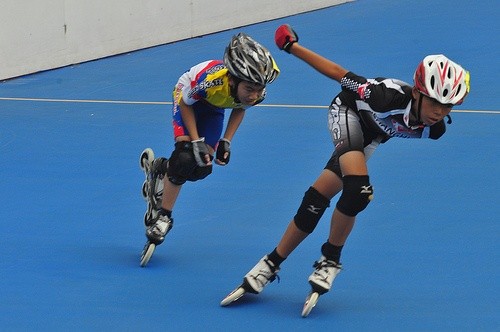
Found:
[219,24,471,318]
[139,32,280,267]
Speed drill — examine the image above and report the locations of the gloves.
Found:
[274,24,299,54]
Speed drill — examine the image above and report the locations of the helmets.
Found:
[413,54,470,105]
[224,32,280,88]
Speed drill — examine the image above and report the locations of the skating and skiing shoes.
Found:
[140,207,173,266]
[301,256,343,317]
[140,148,170,226]
[220,254,280,306]
